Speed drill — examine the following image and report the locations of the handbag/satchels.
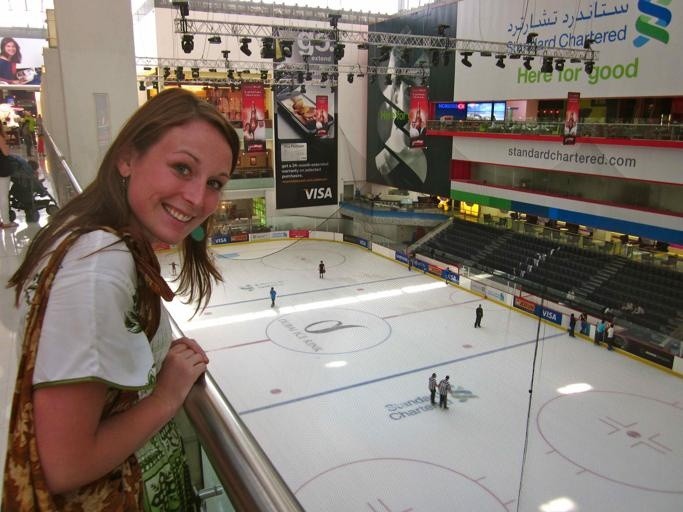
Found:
[0,225,201,511]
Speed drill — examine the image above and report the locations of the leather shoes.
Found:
[0,222,19,227]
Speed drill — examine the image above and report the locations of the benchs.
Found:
[413,216,683,348]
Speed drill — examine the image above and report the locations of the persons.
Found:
[0,37,47,228]
[568,313,576,337]
[427,195,439,208]
[355,187,381,206]
[646,102,659,138]
[506,245,561,289]
[578,311,587,334]
[408,246,451,284]
[270,287,276,307]
[169,262,178,276]
[319,260,325,278]
[474,304,483,327]
[0,88,238,511]
[594,300,645,350]
[428,372,439,404]
[437,376,452,409]
[567,289,575,296]
[620,233,670,252]
[513,211,518,218]
[209,253,216,267]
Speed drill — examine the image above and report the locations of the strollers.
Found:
[6,155,59,222]
